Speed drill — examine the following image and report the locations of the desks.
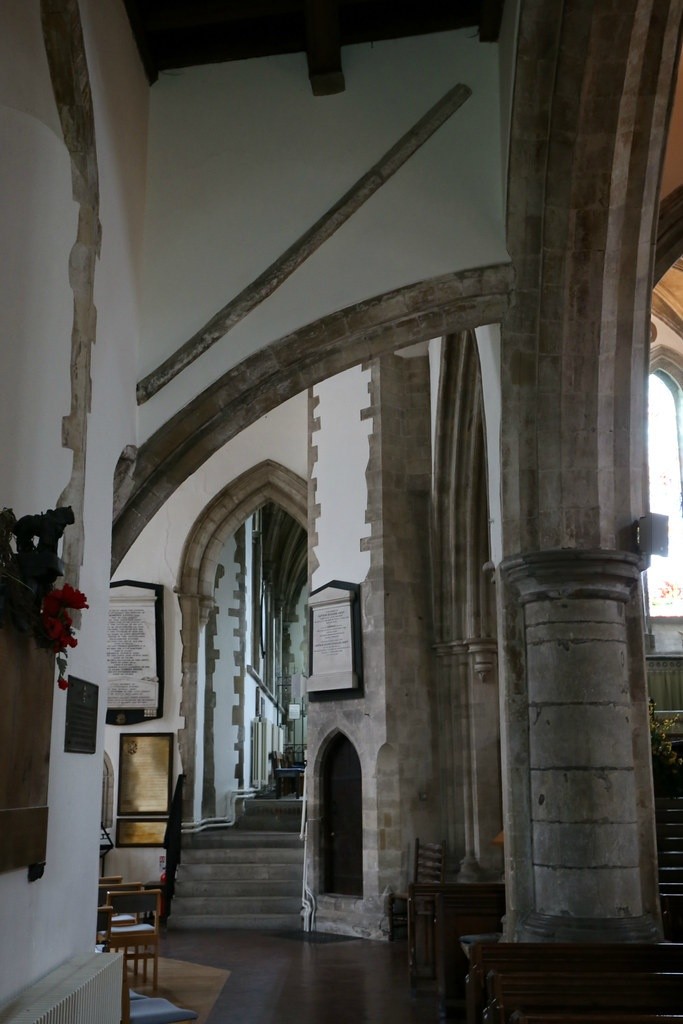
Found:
[408,883,506,1016]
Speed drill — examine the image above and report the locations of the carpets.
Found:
[111,949,231,1024]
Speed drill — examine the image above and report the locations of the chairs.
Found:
[388,836,446,942]
[96,876,199,1024]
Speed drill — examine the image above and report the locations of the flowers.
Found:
[40,583,90,692]
[649,703,683,774]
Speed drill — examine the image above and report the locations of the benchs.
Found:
[268,750,305,799]
